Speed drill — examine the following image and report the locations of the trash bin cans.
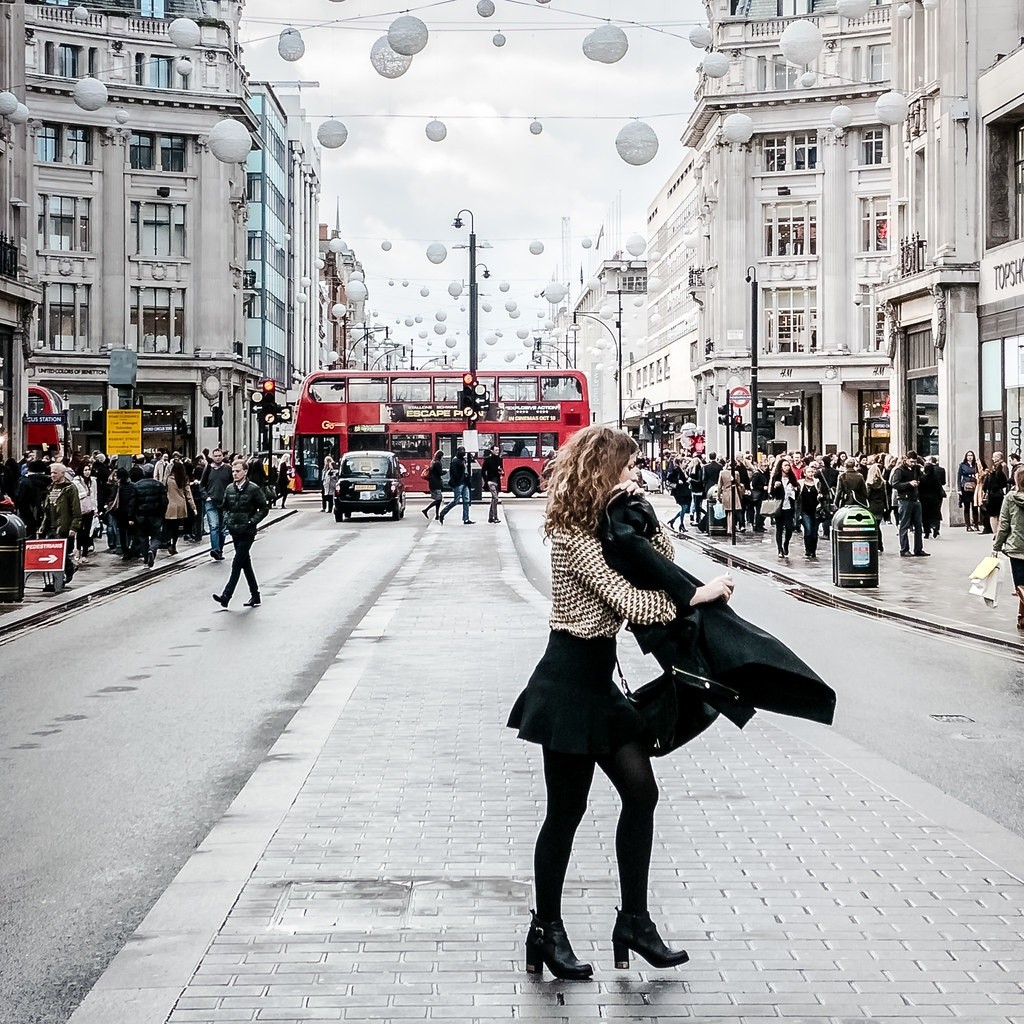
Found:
[0,511,26,604]
[829,505,879,588]
[707,484,731,536]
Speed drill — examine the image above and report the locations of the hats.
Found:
[163,454,169,460]
[254,451,258,456]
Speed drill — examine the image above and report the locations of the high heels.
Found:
[528,911,594,980]
[613,908,689,968]
[666,519,689,532]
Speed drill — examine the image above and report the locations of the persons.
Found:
[506,426,735,979]
[0,449,339,584]
[973,458,992,534]
[1007,453,1024,486]
[982,464,1007,534]
[635,451,946,559]
[514,441,530,457]
[958,451,980,531]
[212,459,269,608]
[991,451,1009,480]
[440,447,476,524]
[991,464,1024,630]
[481,445,505,523]
[422,450,447,520]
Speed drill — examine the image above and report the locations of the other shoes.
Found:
[438,515,445,525]
[243,598,261,607]
[775,546,822,560]
[211,550,225,560]
[922,527,991,539]
[167,547,178,555]
[321,509,332,513]
[489,518,502,524]
[421,510,440,520]
[184,534,201,542]
[213,594,229,608]
[66,546,97,566]
[105,548,122,555]
[143,550,153,570]
[901,549,931,558]
[460,520,475,525]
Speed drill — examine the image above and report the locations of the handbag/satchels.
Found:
[968,551,1003,607]
[80,482,91,514]
[666,471,681,492]
[712,499,726,520]
[828,487,837,500]
[421,468,428,481]
[263,480,277,500]
[761,495,783,518]
[626,673,718,755]
[964,481,977,492]
